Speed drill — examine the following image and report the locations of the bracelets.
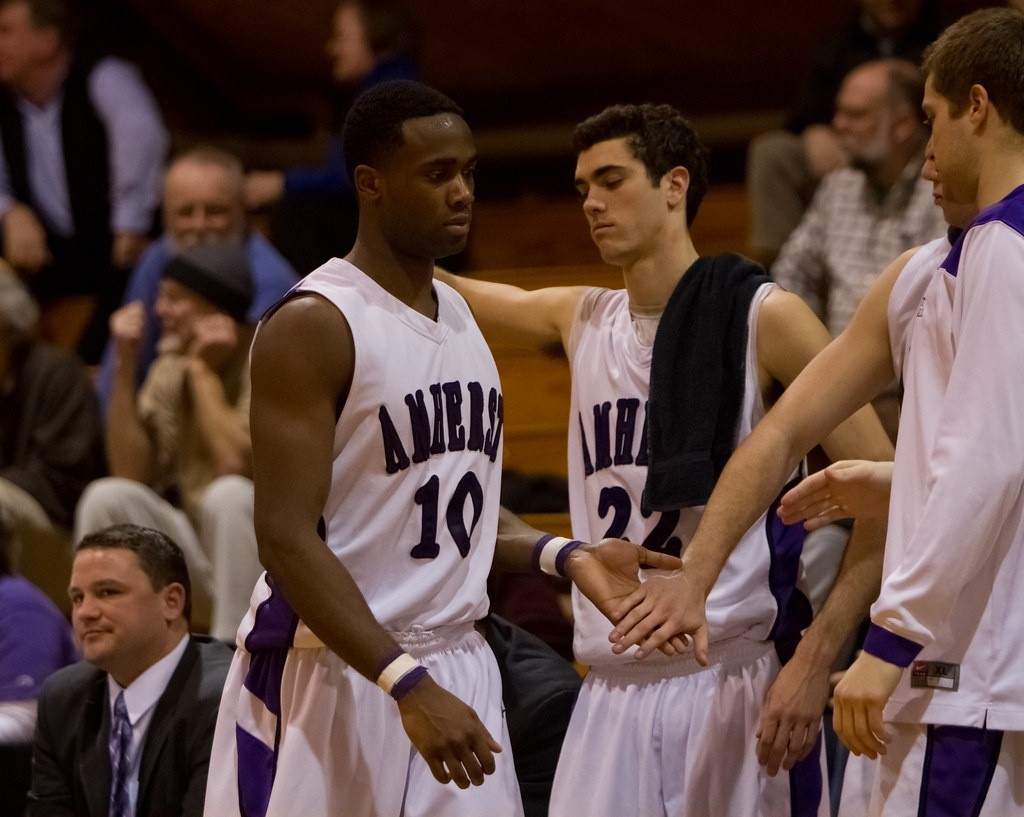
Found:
[532,534,586,578]
[370,650,427,700]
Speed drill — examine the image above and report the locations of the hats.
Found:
[159,239,258,323]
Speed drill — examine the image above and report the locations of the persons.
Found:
[27,526,234,817]
[0,256,114,704]
[0,0,173,365]
[435,103,896,817]
[746,0,1024,272]
[610,8,1024,817]
[239,0,476,283]
[251,78,689,817]
[75,145,304,653]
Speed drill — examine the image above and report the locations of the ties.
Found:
[108,691,136,817]
